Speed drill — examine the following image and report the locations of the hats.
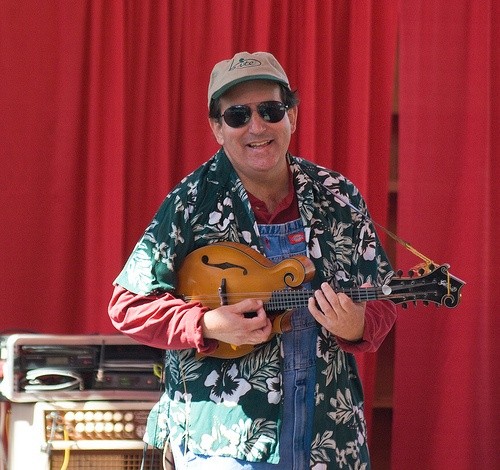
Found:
[207,52,292,108]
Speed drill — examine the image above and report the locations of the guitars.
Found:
[176,241,467,360]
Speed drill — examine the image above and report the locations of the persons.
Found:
[109,52,396,470]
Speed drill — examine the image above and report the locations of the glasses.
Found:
[217,100,291,129]
